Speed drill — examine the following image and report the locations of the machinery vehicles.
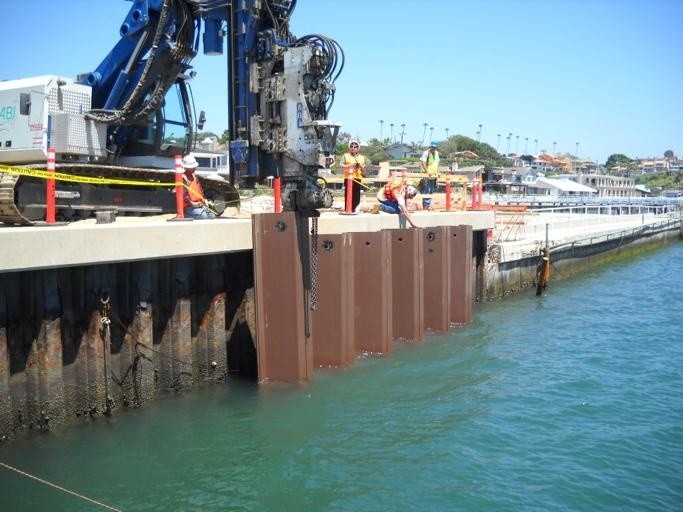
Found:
[0,1,340,216]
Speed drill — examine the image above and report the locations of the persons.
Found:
[339,139,366,212]
[374,183,418,227]
[420,141,440,210]
[182,154,216,220]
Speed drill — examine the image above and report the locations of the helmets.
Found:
[430,141,438,148]
[181,155,199,169]
[347,140,360,151]
[406,185,418,198]
